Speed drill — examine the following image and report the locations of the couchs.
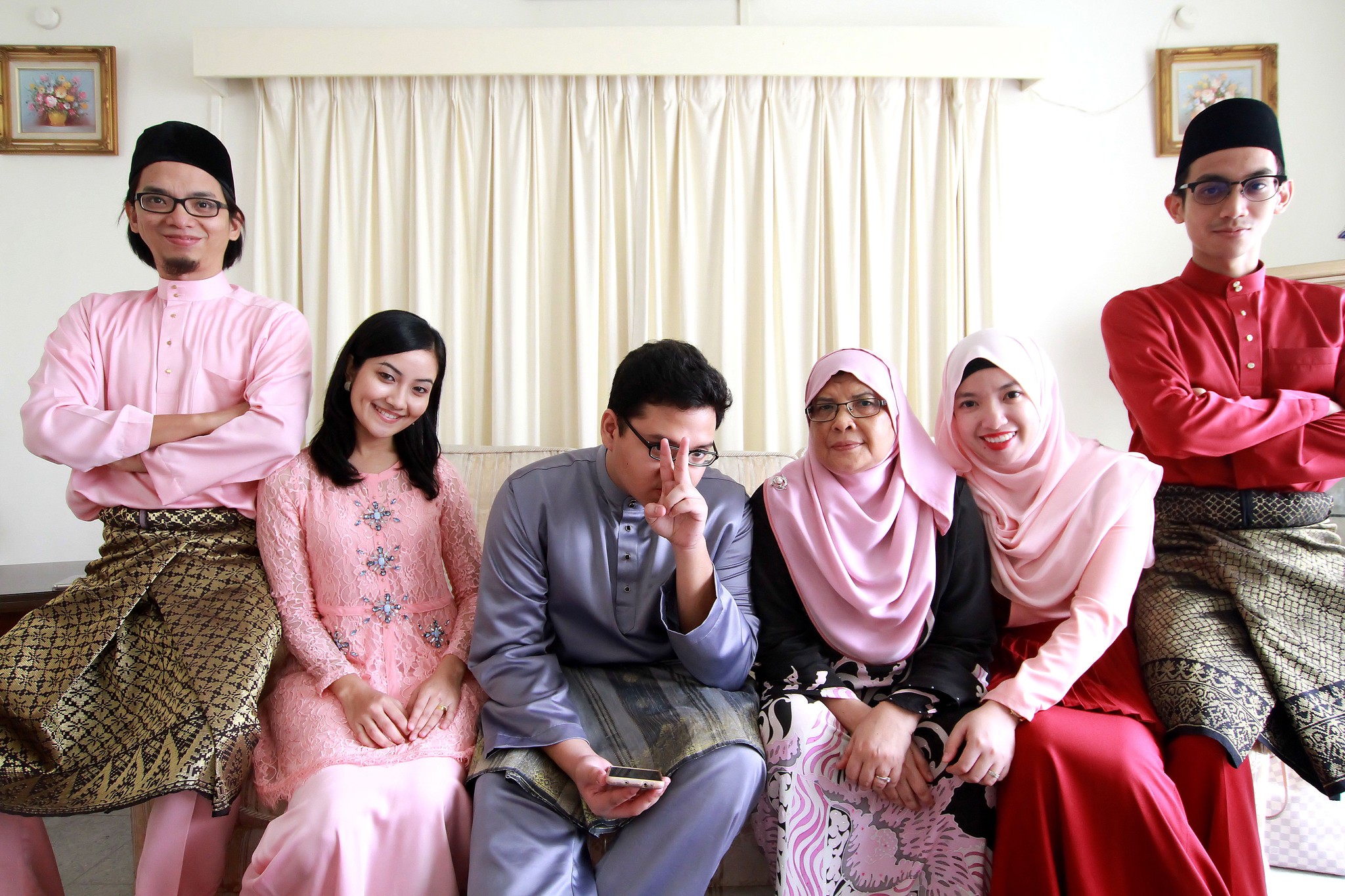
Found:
[131,443,1272,896]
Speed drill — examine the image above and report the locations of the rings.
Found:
[436,705,448,713]
[988,769,1000,779]
[875,774,891,783]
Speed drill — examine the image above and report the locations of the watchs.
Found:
[1008,708,1026,722]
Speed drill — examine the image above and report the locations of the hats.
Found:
[130,120,235,202]
[1176,98,1285,179]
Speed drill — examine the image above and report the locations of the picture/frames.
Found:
[0,44,121,156]
[1154,42,1280,158]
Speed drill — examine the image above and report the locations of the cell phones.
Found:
[604,764,664,787]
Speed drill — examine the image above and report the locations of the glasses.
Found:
[132,192,228,218]
[1178,174,1287,205]
[621,419,719,467]
[805,398,887,422]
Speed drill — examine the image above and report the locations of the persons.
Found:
[932,327,1232,896]
[1100,97,1345,896]
[467,340,768,895]
[745,348,996,896]
[0,122,314,896]
[239,309,486,896]
